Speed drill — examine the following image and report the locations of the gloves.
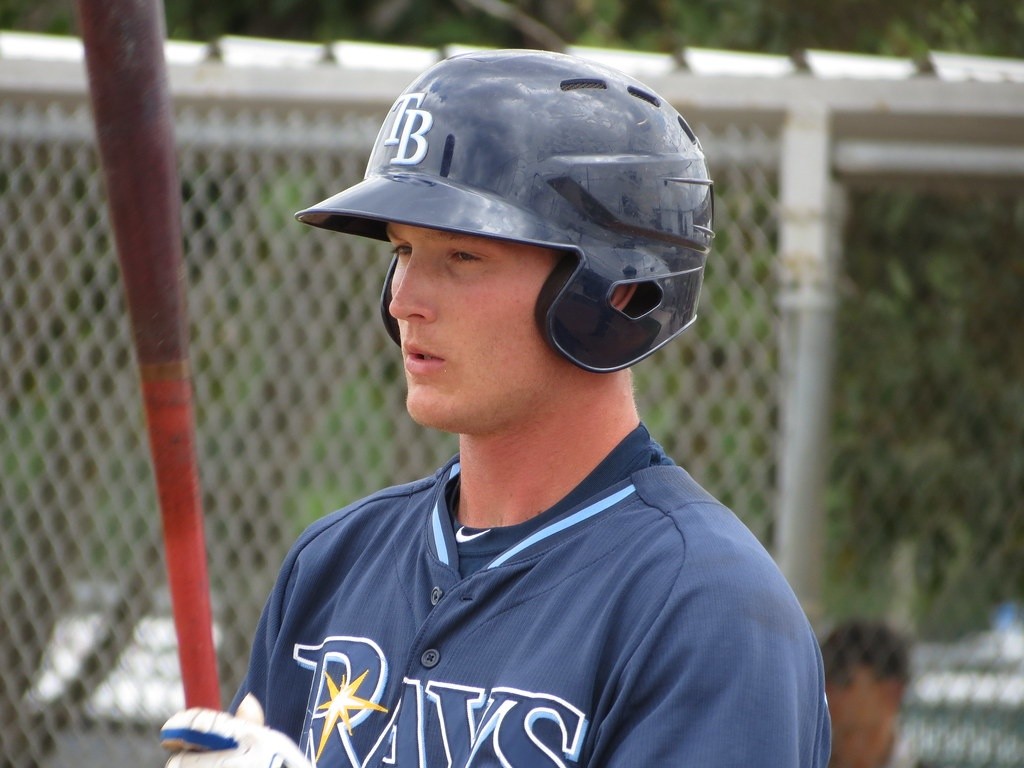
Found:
[160,707,314,768]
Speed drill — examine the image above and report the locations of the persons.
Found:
[153,47,836,767]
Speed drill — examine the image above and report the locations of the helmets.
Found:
[294,53,717,374]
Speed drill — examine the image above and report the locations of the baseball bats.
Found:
[69,0,225,747]
